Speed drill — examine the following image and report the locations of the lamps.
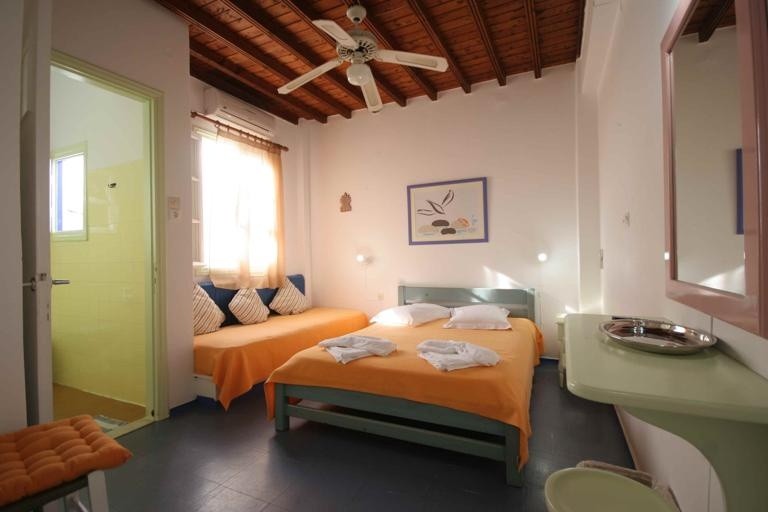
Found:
[345,50,373,87]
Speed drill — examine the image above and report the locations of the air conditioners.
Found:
[201,87,276,141]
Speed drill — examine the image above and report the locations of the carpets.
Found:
[93,414,127,433]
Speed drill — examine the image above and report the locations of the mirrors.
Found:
[659,0,768,339]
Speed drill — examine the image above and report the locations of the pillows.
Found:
[445,304,512,330]
[267,277,311,315]
[193,281,226,334]
[227,281,269,325]
[370,303,449,328]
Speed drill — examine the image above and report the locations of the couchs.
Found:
[192,274,367,408]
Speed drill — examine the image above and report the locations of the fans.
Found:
[278,0,448,116]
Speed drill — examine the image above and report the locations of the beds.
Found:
[264,286,541,488]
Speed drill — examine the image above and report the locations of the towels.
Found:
[416,340,500,372]
[318,334,397,365]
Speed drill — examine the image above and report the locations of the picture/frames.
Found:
[407,177,489,245]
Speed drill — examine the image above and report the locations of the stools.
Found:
[0,416,133,512]
[543,468,673,512]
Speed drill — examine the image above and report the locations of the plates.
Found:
[599,318,717,356]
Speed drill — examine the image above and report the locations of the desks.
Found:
[555,312,566,389]
